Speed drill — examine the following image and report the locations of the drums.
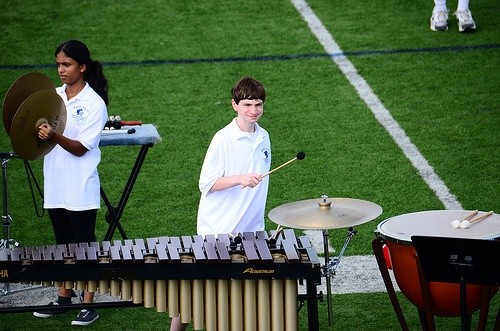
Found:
[377,210,500,318]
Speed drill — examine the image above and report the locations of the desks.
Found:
[99,123,160,241]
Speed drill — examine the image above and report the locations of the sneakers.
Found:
[455,9,476,33]
[430,7,448,31]
[71,309,99,325]
[33,302,71,318]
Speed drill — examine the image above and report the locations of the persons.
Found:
[32,40,109,326]
[430,0,477,32]
[169,77,271,331]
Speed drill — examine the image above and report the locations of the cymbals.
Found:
[2,72,67,160]
[268,195,383,229]
